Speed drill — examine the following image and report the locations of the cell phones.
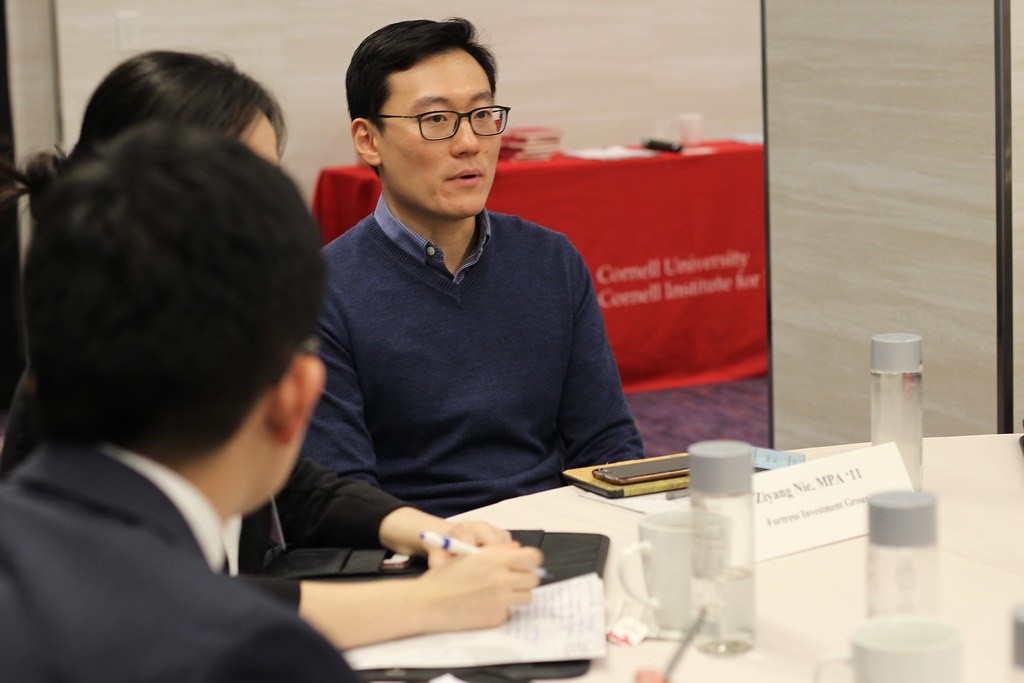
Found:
[592,456,691,485]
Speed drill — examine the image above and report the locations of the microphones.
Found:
[642,138,681,153]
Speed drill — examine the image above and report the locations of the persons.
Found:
[0,122,358,683]
[300,18,644,519]
[0,51,543,653]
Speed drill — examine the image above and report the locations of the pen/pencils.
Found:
[666,604,709,679]
[418,532,557,582]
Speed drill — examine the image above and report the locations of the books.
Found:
[496,128,559,162]
[563,453,690,499]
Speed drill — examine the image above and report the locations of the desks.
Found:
[311,140,766,400]
[375,431,1024,682]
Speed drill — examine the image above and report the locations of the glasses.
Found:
[364,105,511,141]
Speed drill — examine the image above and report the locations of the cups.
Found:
[690,441,757,655]
[871,333,923,492]
[679,114,701,144]
[616,511,729,632]
[867,492,937,620]
[815,615,963,683]
